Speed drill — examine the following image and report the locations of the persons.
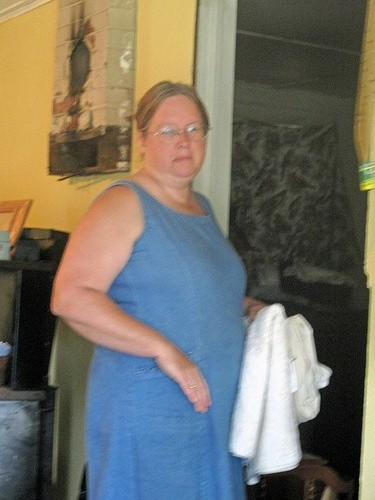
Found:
[49,80,277,500]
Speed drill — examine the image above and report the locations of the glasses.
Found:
[153,120,210,141]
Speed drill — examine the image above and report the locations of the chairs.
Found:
[258,453,355,500]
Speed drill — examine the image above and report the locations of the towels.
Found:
[228,303,332,485]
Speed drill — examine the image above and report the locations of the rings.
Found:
[189,384,199,388]
